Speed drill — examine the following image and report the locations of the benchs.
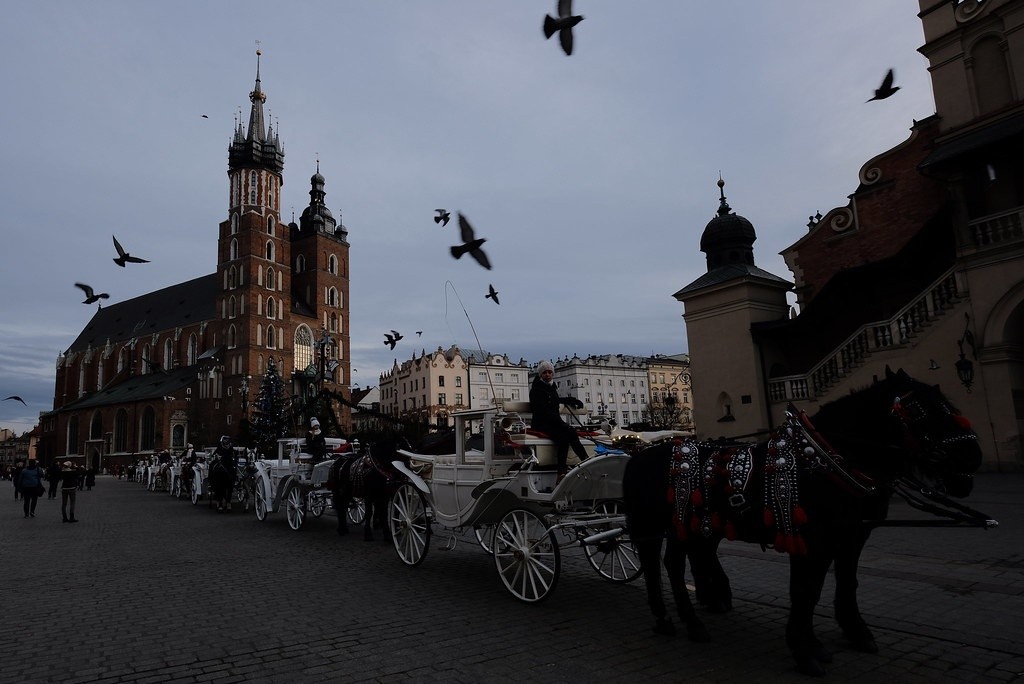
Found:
[288,435,347,476]
[496,409,613,474]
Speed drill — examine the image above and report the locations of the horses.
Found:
[628,369,984,676]
[242,447,260,505]
[328,437,415,543]
[160,461,173,491]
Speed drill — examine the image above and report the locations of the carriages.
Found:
[389,363,999,676]
[128,448,261,513]
[254,429,415,542]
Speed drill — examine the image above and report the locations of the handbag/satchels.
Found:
[38,482,45,496]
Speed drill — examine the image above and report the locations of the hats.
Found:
[310,417,320,428]
[538,360,554,377]
[187,443,193,448]
[64,461,72,467]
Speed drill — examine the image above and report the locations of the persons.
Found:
[307,416,326,464]
[46,460,62,500]
[180,443,196,464]
[530,361,589,479]
[77,464,86,491]
[212,435,232,457]
[497,418,530,454]
[60,461,79,522]
[127,468,134,481]
[12,462,24,501]
[159,449,172,464]
[112,468,122,479]
[20,459,45,519]
[85,466,95,490]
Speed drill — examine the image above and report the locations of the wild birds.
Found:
[113,234,151,267]
[383,334,396,350]
[416,331,422,336]
[543,1,583,56]
[1,396,27,406]
[486,284,500,305]
[434,208,450,226]
[353,383,359,387]
[74,283,109,304]
[391,330,403,341]
[866,70,901,102]
[451,213,492,271]
[354,369,357,372]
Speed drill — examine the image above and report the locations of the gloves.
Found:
[575,399,583,409]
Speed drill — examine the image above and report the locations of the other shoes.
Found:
[25,515,28,518]
[556,474,565,484]
[30,512,35,517]
[69,519,78,523]
[63,520,69,522]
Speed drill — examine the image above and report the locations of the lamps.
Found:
[952,309,980,394]
